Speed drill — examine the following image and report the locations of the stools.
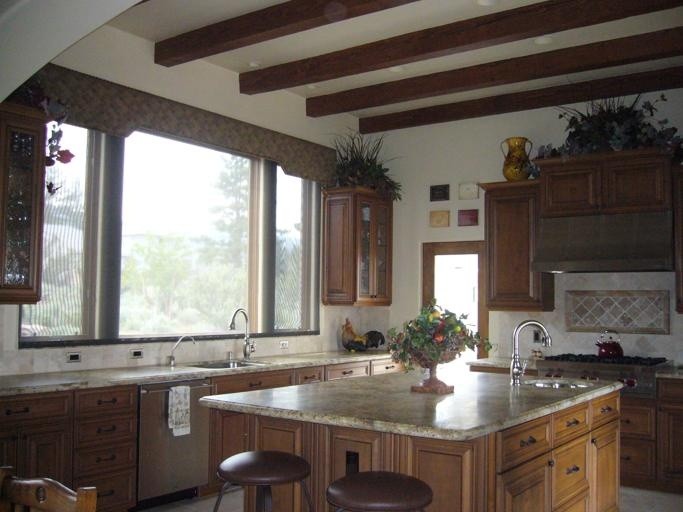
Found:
[324,470,433,511]
[210,448,315,511]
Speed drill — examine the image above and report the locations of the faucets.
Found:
[170,335,195,357]
[230,308,249,341]
[512,320,551,355]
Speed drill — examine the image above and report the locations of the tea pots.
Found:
[595,330,623,358]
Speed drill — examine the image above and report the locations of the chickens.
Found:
[342,318,385,352]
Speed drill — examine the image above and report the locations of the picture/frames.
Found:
[429,182,479,228]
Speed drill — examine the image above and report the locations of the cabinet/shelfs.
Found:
[0,101,49,302]
[529,149,672,217]
[486,387,620,512]
[477,180,555,312]
[620,376,683,494]
[209,408,486,511]
[0,384,139,511]
[320,185,392,307]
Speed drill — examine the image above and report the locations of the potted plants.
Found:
[327,127,403,202]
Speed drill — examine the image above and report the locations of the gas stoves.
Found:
[537,352,673,399]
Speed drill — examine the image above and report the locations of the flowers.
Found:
[522,88,683,161]
[19,68,73,194]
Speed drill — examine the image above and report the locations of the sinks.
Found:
[525,379,595,391]
[184,360,269,368]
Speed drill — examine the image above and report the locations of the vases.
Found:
[602,121,632,151]
[11,85,45,107]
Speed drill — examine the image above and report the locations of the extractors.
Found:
[531,211,676,274]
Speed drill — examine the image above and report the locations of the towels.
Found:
[167,386,191,437]
